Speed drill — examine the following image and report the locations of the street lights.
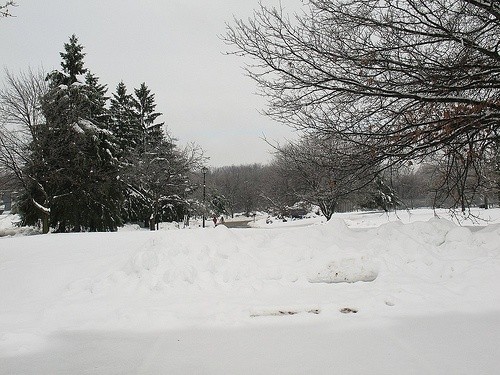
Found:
[200,166,209,228]
